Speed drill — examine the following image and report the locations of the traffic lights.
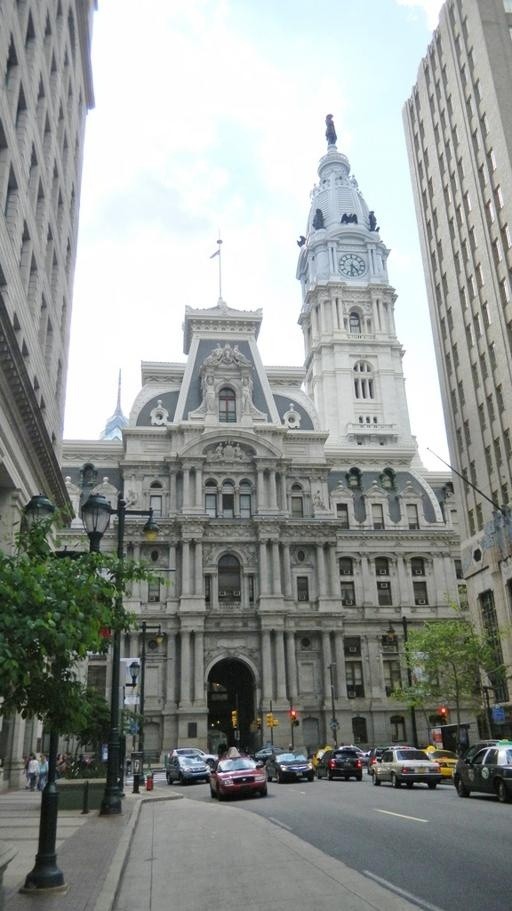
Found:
[267,713,273,727]
[440,706,447,717]
[232,711,240,728]
[290,711,296,724]
[294,720,299,726]
[257,718,262,728]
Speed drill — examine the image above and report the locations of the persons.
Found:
[23,752,95,792]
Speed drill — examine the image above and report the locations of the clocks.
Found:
[339,253,365,278]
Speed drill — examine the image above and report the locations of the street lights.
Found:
[120,661,142,795]
[16,489,111,895]
[136,617,164,785]
[76,461,157,816]
[386,616,418,747]
[328,661,339,748]
[129,752,141,794]
